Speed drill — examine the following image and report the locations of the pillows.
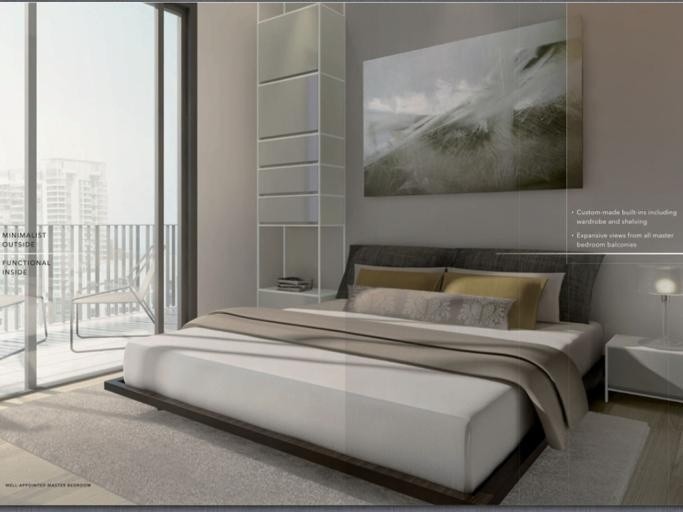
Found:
[342,263,566,331]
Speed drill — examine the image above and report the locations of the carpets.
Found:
[0,383,651,505]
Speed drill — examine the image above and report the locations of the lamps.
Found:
[638,263,683,350]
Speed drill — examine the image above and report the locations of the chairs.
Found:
[0,293,48,361]
[70,243,164,352]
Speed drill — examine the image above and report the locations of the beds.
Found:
[104,244,605,505]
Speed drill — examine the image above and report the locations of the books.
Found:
[276,276,312,293]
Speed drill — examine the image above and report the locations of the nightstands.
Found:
[605,333,683,405]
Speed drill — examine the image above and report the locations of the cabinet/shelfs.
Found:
[257,2,346,308]
